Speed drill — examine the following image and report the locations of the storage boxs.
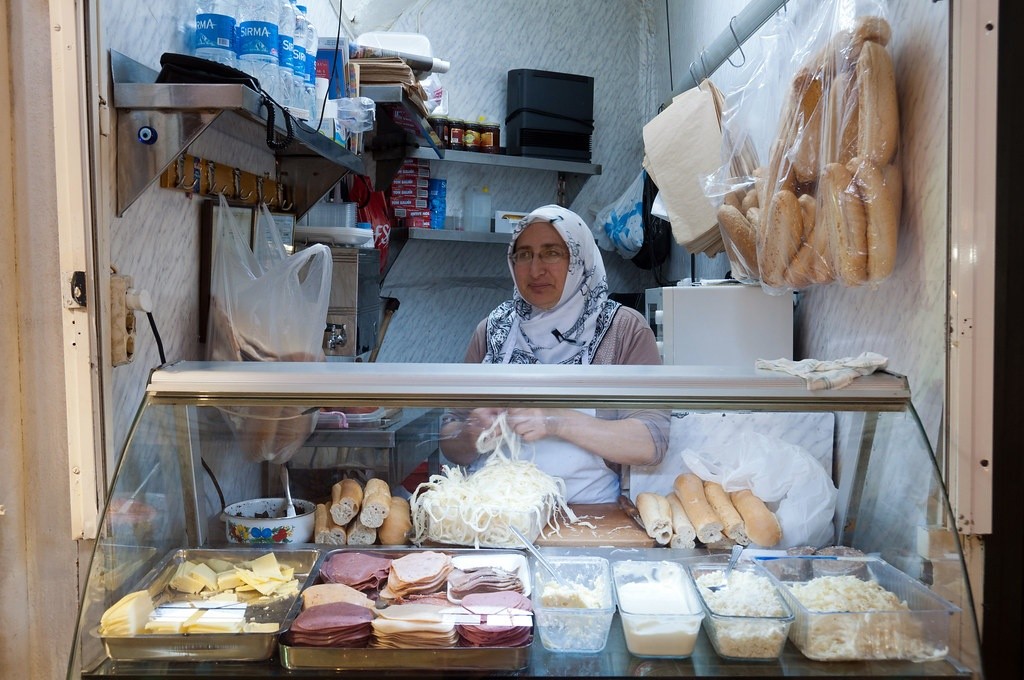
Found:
[386,156,431,230]
[533,545,963,664]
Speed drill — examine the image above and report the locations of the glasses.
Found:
[512,245,571,267]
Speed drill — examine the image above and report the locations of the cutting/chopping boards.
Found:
[419,502,656,548]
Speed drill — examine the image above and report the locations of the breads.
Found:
[717,16,898,289]
[312,477,413,546]
[235,353,322,462]
[636,473,782,551]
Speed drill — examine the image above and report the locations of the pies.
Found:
[208,297,281,425]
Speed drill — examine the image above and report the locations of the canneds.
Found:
[428,116,501,154]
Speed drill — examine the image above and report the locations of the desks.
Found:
[261,402,444,500]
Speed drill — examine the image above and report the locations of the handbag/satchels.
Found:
[212,192,332,362]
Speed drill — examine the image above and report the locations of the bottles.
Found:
[464,185,492,232]
[194,0,318,127]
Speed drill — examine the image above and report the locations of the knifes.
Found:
[619,495,646,530]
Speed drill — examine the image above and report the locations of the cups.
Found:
[453,209,464,231]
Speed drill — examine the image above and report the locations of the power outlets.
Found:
[110,273,137,368]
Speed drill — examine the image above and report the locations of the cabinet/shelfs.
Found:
[405,145,603,259]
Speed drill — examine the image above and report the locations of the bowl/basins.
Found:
[310,202,358,227]
[531,556,616,654]
[689,562,795,662]
[611,559,706,659]
[219,498,317,543]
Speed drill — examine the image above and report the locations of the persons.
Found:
[437,204,670,503]
